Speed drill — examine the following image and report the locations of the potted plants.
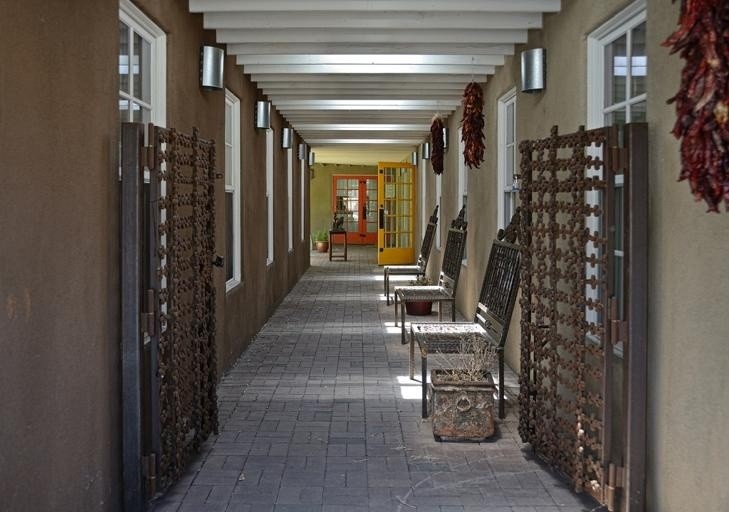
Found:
[405,277,433,315]
[429,326,497,444]
[315,230,329,253]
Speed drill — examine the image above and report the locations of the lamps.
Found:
[256,99,270,128]
[442,128,449,148]
[412,151,418,165]
[308,151,314,166]
[299,144,307,161]
[521,47,545,91]
[200,46,224,91]
[422,143,430,159]
[282,128,294,149]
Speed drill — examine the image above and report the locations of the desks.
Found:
[329,230,347,261]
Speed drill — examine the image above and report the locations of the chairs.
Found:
[395,203,467,344]
[383,205,442,305]
[410,206,522,420]
